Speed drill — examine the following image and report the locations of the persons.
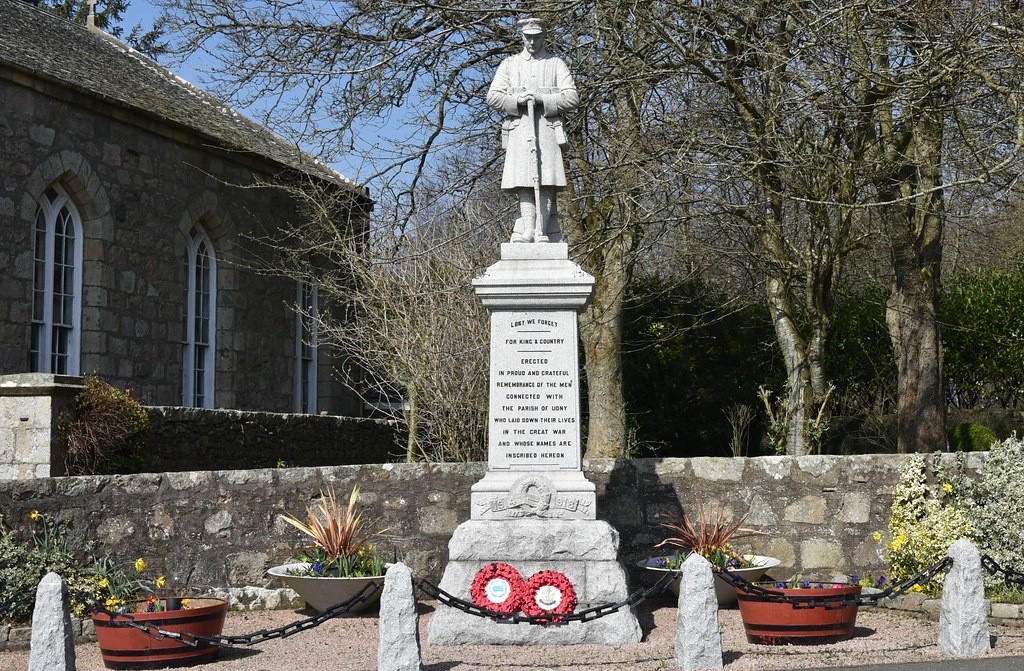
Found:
[486,17,579,243]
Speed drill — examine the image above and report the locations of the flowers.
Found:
[751,573,858,590]
[645,490,758,571]
[276,481,387,578]
[104,593,191,615]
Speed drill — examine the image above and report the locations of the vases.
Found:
[266,562,396,617]
[635,554,782,609]
[91,596,229,670]
[735,581,862,646]
